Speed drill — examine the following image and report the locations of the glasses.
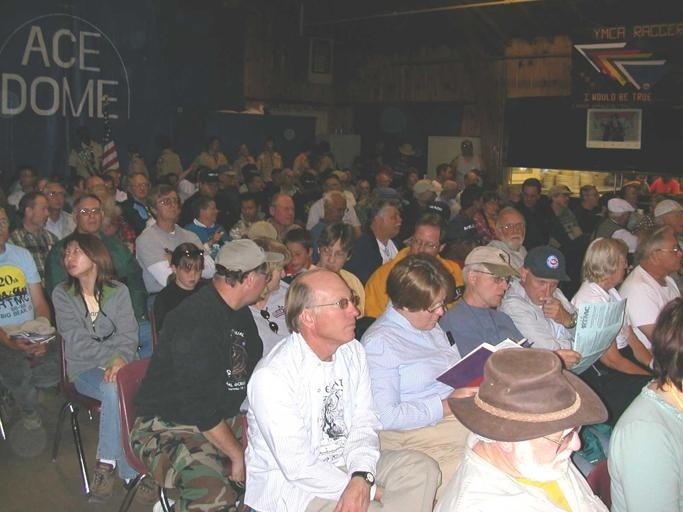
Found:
[92,330,115,341]
[261,310,278,332]
[49,192,100,215]
[544,426,581,455]
[505,224,524,229]
[135,183,179,205]
[657,248,678,253]
[319,296,359,309]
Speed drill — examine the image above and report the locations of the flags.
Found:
[99,96,121,175]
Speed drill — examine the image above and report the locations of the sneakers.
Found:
[121,479,159,505]
[89,460,116,503]
[22,412,41,432]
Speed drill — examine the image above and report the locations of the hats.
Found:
[465,246,521,277]
[608,198,635,212]
[214,239,284,272]
[413,180,437,193]
[654,199,683,217]
[525,246,570,281]
[447,348,608,442]
[200,171,220,182]
[548,184,572,198]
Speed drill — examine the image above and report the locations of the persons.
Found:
[0,127,683,512]
[601,114,624,142]
